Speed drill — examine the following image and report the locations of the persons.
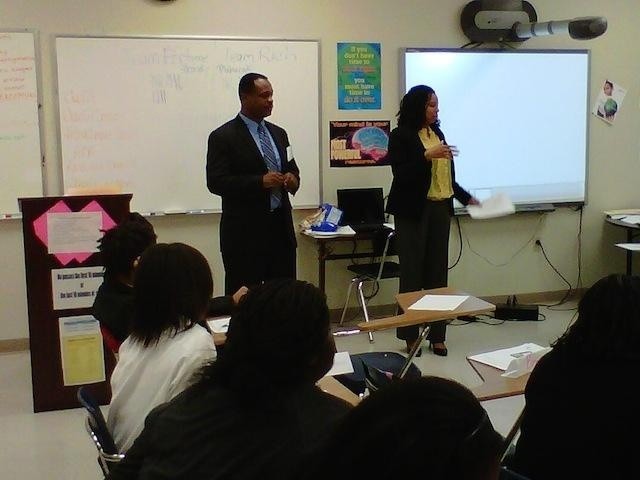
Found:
[508,270,640,480]
[103,277,355,480]
[203,71,305,294]
[90,211,158,344]
[106,240,218,456]
[292,373,508,480]
[383,83,482,359]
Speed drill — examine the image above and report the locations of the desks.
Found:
[464,376,559,466]
[357,284,494,374]
[301,226,402,295]
[207,311,233,347]
[604,214,640,277]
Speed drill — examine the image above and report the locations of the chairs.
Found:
[78,383,133,479]
[338,230,399,343]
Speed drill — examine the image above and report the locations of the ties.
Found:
[256,125,283,210]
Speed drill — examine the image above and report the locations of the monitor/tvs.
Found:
[336,187,386,227]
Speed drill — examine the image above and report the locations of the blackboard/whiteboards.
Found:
[50,34,324,217]
[1,28,48,219]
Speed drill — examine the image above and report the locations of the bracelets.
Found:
[425,149,432,162]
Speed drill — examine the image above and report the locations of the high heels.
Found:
[428,339,448,356]
[406,338,423,358]
